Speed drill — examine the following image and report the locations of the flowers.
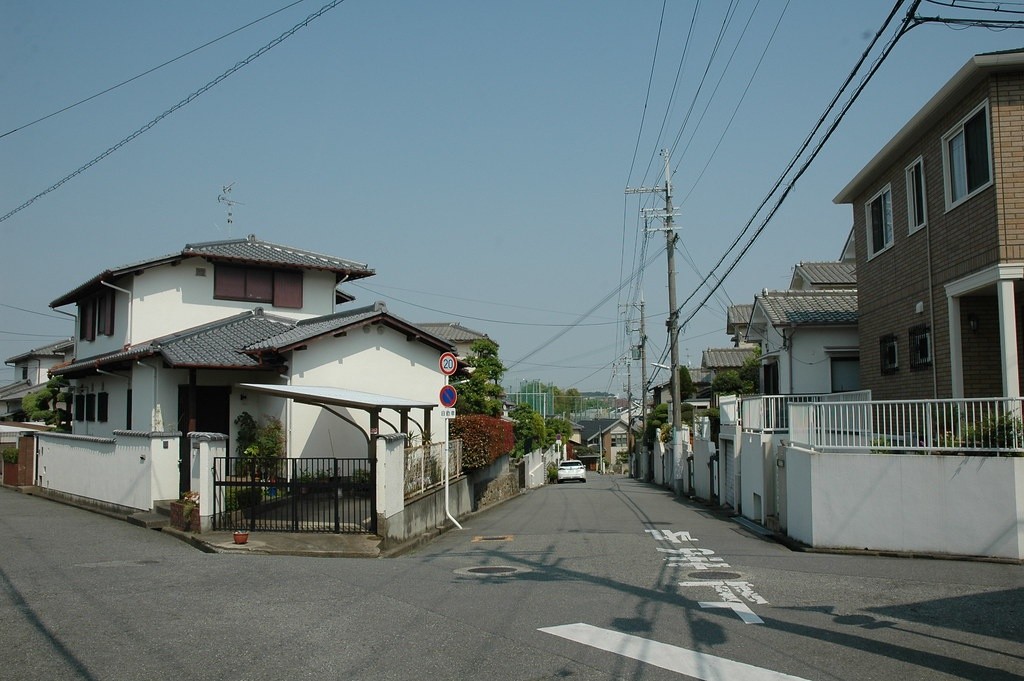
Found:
[179,491,200,524]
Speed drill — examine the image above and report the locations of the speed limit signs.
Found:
[438,352,456,376]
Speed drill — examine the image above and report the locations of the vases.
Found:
[233,532,248,544]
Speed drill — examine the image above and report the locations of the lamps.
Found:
[970,315,979,334]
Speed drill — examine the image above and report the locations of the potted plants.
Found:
[299,467,313,494]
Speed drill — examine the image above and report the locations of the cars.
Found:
[557,459,587,483]
